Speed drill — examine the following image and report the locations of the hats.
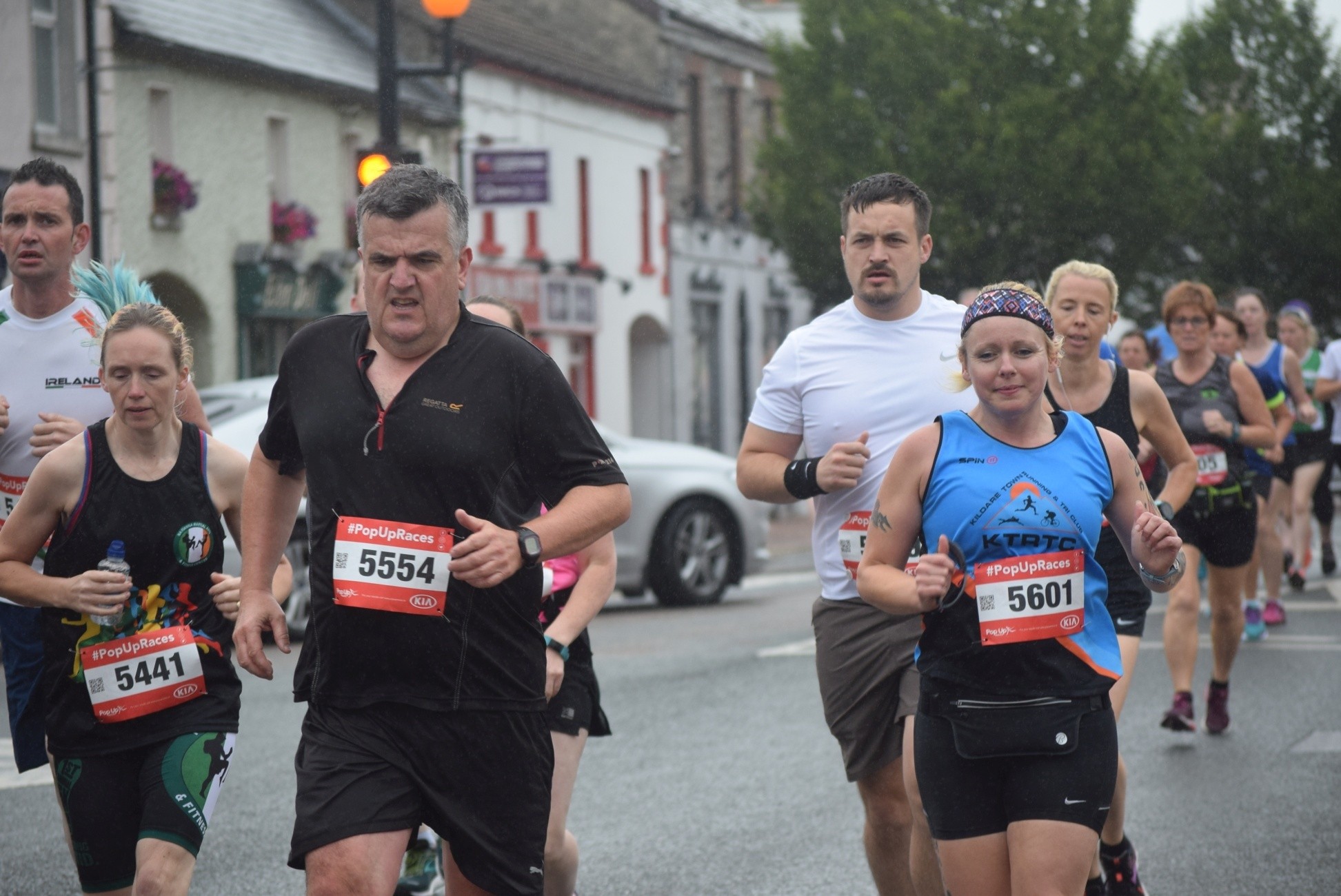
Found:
[1280,299,1311,321]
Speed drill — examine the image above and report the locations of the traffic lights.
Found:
[353,151,422,201]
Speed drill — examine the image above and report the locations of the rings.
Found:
[237,601,241,611]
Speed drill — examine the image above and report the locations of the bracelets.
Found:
[784,456,830,499]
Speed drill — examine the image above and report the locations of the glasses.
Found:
[1170,315,1212,327]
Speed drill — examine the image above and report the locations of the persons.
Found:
[737,173,1341,896]
[0,156,629,896]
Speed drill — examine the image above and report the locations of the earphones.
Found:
[1106,322,1111,334]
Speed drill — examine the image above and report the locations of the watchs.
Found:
[1154,500,1175,521]
[543,635,570,661]
[512,526,543,570]
[1229,420,1240,443]
[1140,559,1179,583]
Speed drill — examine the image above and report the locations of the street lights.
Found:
[369,2,489,188]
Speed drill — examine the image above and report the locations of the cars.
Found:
[197,377,781,645]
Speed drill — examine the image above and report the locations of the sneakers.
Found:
[1244,599,1265,639]
[1101,842,1148,896]
[1263,600,1287,622]
[1206,685,1229,730]
[1160,702,1197,730]
[393,837,445,896]
